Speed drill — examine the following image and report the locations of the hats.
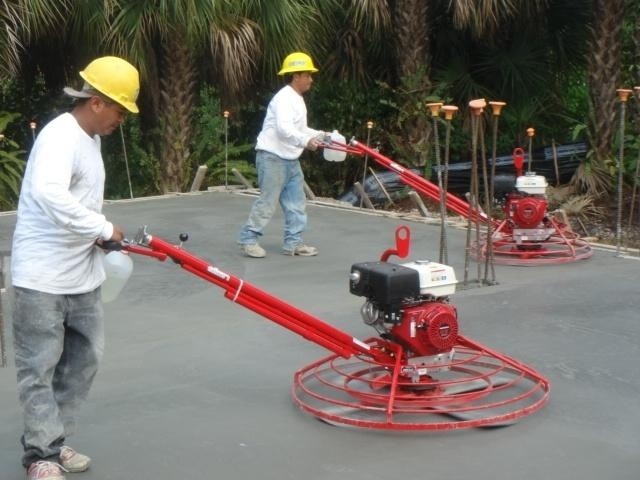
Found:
[63,81,115,105]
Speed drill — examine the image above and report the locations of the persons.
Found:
[10,56,140,480]
[235,52,330,257]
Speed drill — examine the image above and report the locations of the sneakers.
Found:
[284,243,318,256]
[60,445,91,472]
[26,461,66,480]
[238,243,266,257]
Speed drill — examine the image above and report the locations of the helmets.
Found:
[79,55,140,113]
[278,52,319,76]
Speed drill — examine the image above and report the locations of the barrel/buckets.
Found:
[324,128,346,163]
[101,248,134,303]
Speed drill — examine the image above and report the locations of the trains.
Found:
[340,141,590,210]
